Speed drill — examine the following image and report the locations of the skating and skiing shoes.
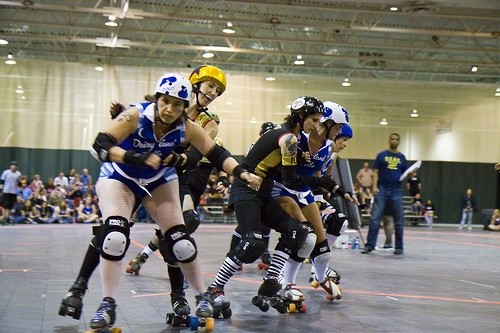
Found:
[126,256,144,276]
[205,286,232,319]
[279,278,308,313]
[87,297,122,332]
[250,278,292,314]
[309,267,342,284]
[57,289,83,320]
[167,293,194,327]
[194,294,216,331]
[310,274,344,303]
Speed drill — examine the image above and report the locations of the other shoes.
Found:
[361,248,372,254]
[393,249,404,253]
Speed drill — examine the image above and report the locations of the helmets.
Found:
[154,73,192,108]
[189,64,226,97]
[337,123,353,139]
[319,101,350,123]
[290,95,325,116]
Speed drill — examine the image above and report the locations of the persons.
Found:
[198,96,360,320]
[130,203,155,223]
[406,172,421,203]
[58,65,227,328]
[88,72,260,333]
[459,188,476,230]
[413,193,422,215]
[0,160,103,225]
[424,199,434,228]
[354,133,413,254]
[487,209,500,231]
[126,112,231,289]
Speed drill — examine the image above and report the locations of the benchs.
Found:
[10,184,438,226]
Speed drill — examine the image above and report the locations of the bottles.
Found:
[355,237,360,248]
[348,237,353,249]
